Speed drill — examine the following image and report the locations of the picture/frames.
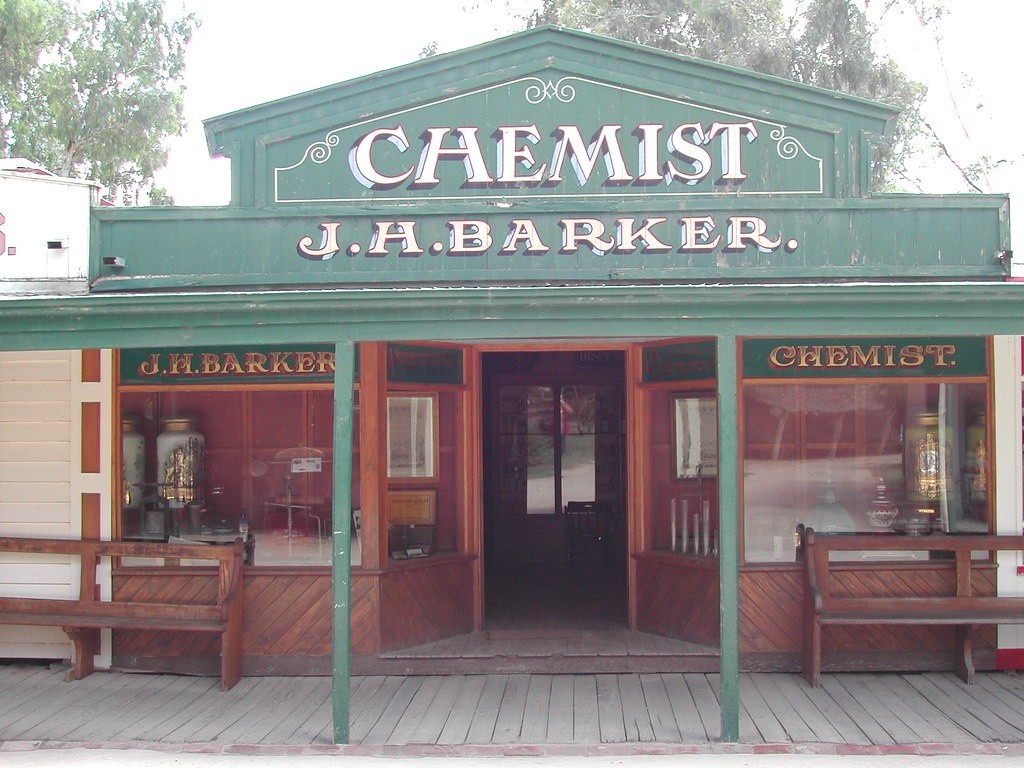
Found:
[387,391,439,484]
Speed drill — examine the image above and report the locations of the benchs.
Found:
[0,536,243,691]
[802,527,1024,687]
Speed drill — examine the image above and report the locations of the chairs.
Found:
[564,502,616,576]
